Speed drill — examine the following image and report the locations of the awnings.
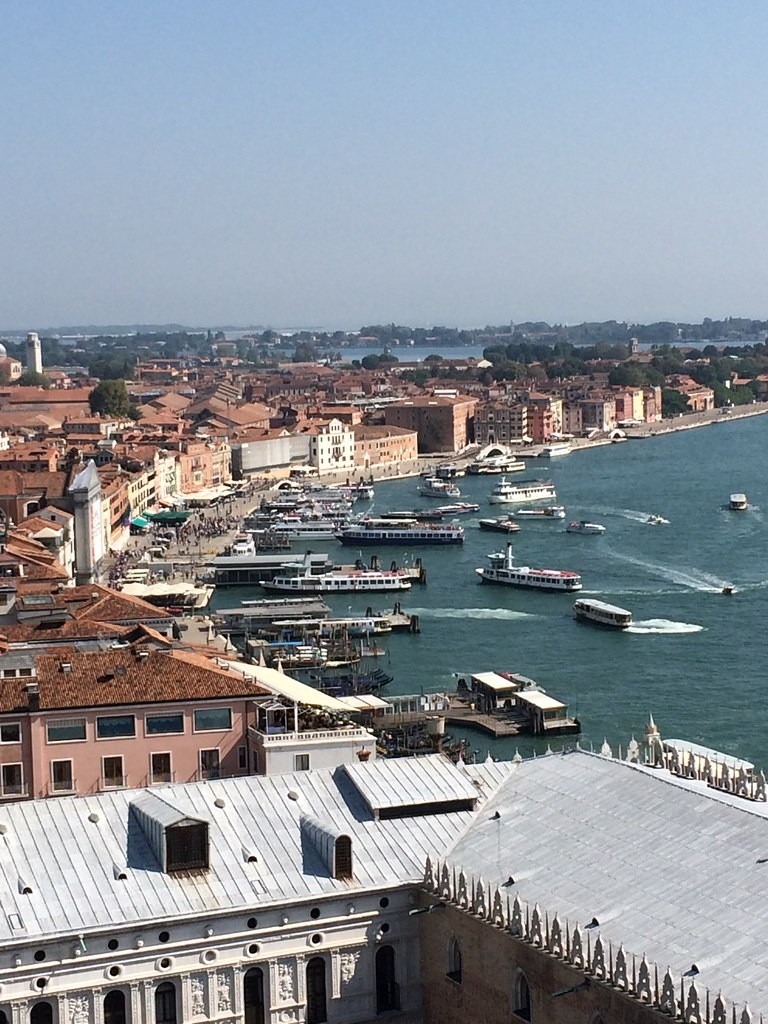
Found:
[131,492,185,530]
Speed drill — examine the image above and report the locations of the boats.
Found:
[379,508,445,522]
[258,561,414,592]
[375,723,486,764]
[478,515,521,535]
[416,475,460,498]
[566,518,606,535]
[475,539,583,592]
[244,476,373,541]
[435,500,480,516]
[730,492,748,513]
[539,444,574,458]
[333,516,466,545]
[196,548,337,586]
[216,595,395,695]
[573,598,634,628]
[421,460,528,480]
[231,533,259,557]
[507,505,566,520]
[485,476,557,504]
[721,585,735,598]
